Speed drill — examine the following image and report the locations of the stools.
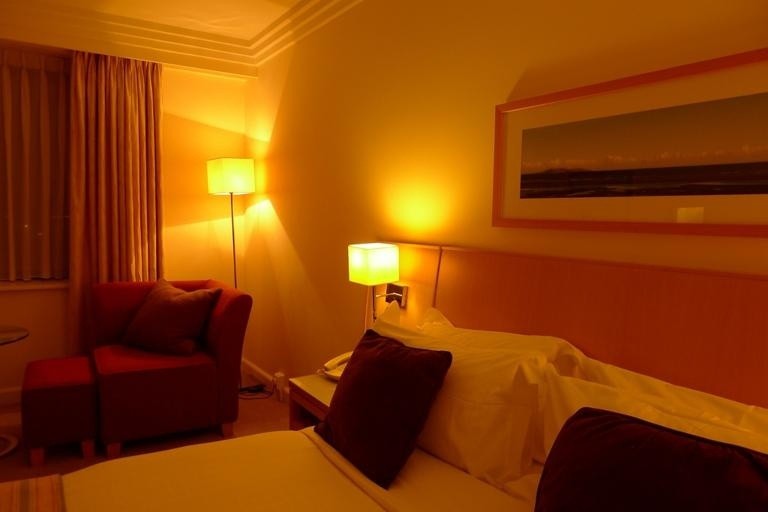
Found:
[20,356,98,465]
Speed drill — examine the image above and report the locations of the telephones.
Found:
[324,351,354,381]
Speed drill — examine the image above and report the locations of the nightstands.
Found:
[289,370,340,430]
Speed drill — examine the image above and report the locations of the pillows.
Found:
[121,276,222,355]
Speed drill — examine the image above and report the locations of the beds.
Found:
[0,241,768,510]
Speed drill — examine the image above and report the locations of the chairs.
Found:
[81,277,253,453]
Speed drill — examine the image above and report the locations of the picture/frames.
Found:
[490,46,768,238]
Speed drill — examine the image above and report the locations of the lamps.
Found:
[205,155,266,394]
[346,242,401,323]
